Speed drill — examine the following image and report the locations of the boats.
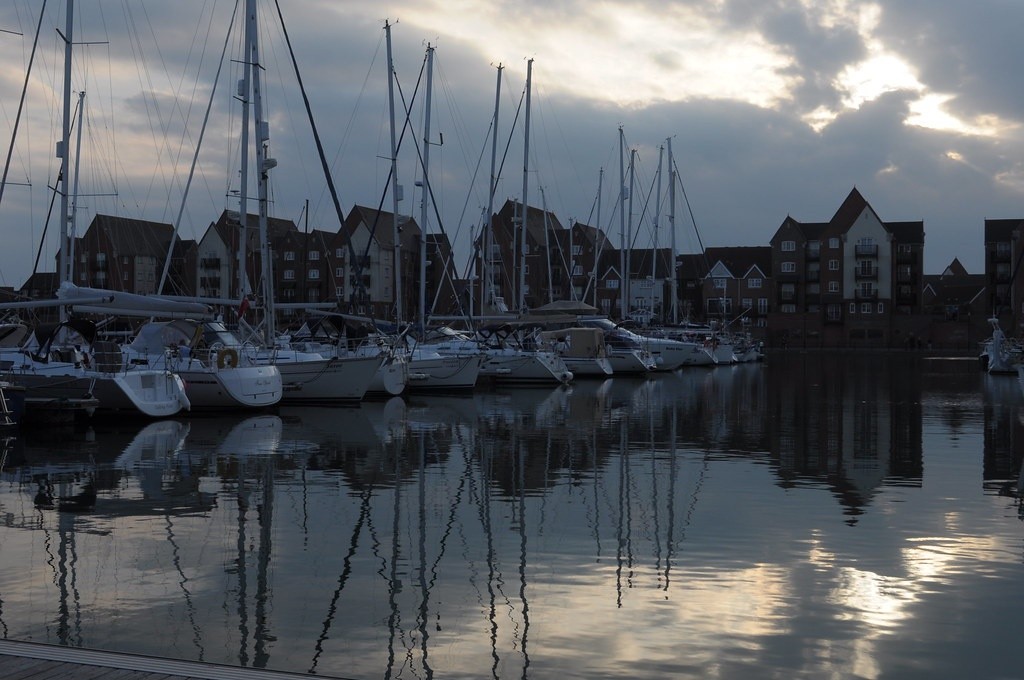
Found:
[978,316,1023,374]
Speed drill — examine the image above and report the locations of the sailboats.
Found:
[1,1,764,419]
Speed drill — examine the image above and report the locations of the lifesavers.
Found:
[218,348,238,368]
